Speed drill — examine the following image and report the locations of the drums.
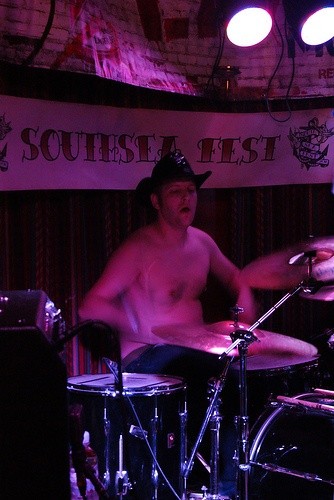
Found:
[246,392,334,500]
[68,373,189,500]
[221,354,321,482]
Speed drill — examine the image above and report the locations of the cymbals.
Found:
[236,237,334,289]
[151,321,312,361]
[300,286,334,301]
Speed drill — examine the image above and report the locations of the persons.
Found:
[78,150,255,455]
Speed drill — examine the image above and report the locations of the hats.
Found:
[135,151,213,199]
